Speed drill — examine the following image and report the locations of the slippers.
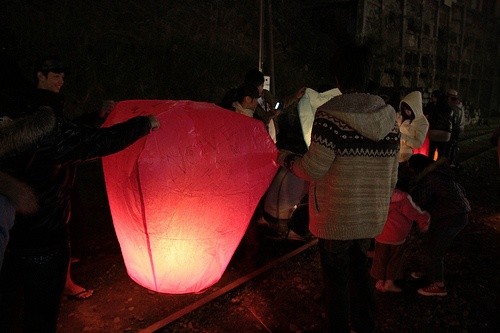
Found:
[62,286,93,299]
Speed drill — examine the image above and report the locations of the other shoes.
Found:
[411,271,427,280]
[417,283,447,296]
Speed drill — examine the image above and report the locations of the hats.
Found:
[446,89,459,98]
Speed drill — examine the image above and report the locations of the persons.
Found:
[370,69,471,296]
[197,71,307,241]
[0,60,159,333]
[277,43,401,333]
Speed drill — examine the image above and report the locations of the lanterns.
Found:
[265,168,307,224]
[99,100,280,294]
[298,88,343,150]
